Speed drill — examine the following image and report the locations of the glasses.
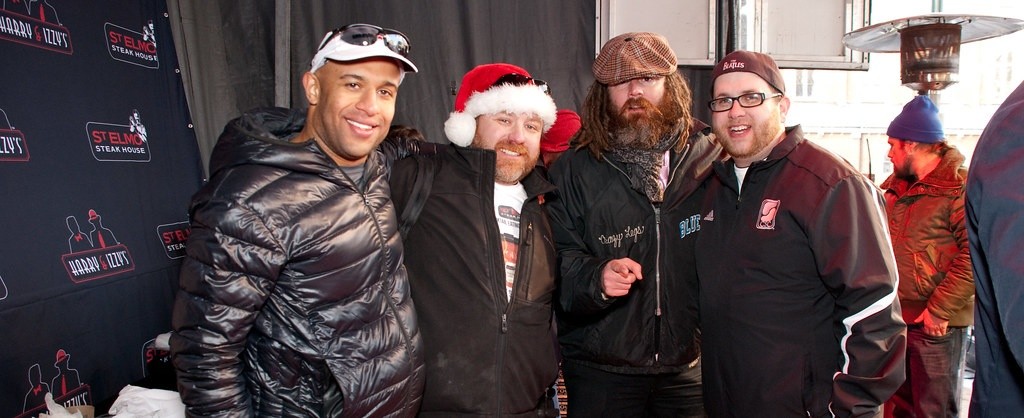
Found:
[486,74,551,95]
[317,24,411,60]
[708,92,782,112]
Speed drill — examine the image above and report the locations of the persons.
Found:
[544,31,730,418]
[879,94,974,418]
[169,22,583,418]
[692,50,906,418]
[965,79,1024,418]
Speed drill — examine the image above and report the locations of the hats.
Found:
[444,64,557,147]
[710,51,786,99]
[887,95,944,143]
[310,30,418,73]
[541,109,581,152]
[592,32,678,86]
